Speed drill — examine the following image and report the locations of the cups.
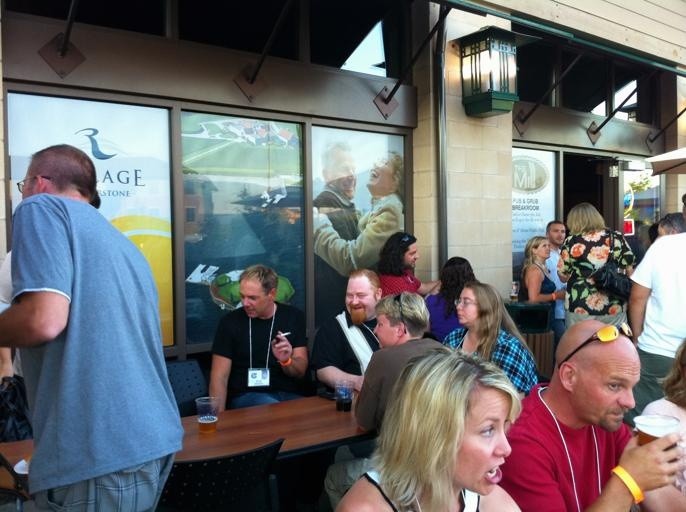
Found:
[196,397,218,432]
[633,415,680,453]
[335,380,354,411]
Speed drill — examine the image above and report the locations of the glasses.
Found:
[393,294,407,334]
[454,300,480,305]
[17,175,50,193]
[558,322,634,369]
[399,233,408,245]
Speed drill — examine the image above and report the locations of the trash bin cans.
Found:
[503,301,555,375]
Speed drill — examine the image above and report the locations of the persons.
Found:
[375,232,439,296]
[312,150,404,278]
[0,190,101,388]
[337,346,522,512]
[442,282,538,393]
[309,269,382,391]
[557,202,637,331]
[623,193,686,426]
[425,257,476,342]
[519,237,566,312]
[658,212,686,236]
[491,318,685,512]
[0,144,185,511]
[209,265,307,412]
[546,220,567,345]
[324,290,442,511]
[627,225,650,264]
[636,339,685,490]
[311,140,359,329]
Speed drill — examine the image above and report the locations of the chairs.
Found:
[168,359,213,414]
[157,437,287,506]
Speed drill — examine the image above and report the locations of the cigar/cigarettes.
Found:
[280,332,291,337]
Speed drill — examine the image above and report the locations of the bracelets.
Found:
[610,466,644,504]
[279,358,292,367]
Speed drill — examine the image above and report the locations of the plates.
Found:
[15,455,31,473]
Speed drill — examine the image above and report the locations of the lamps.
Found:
[449,25,542,119]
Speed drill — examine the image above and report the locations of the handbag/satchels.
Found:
[593,227,633,300]
